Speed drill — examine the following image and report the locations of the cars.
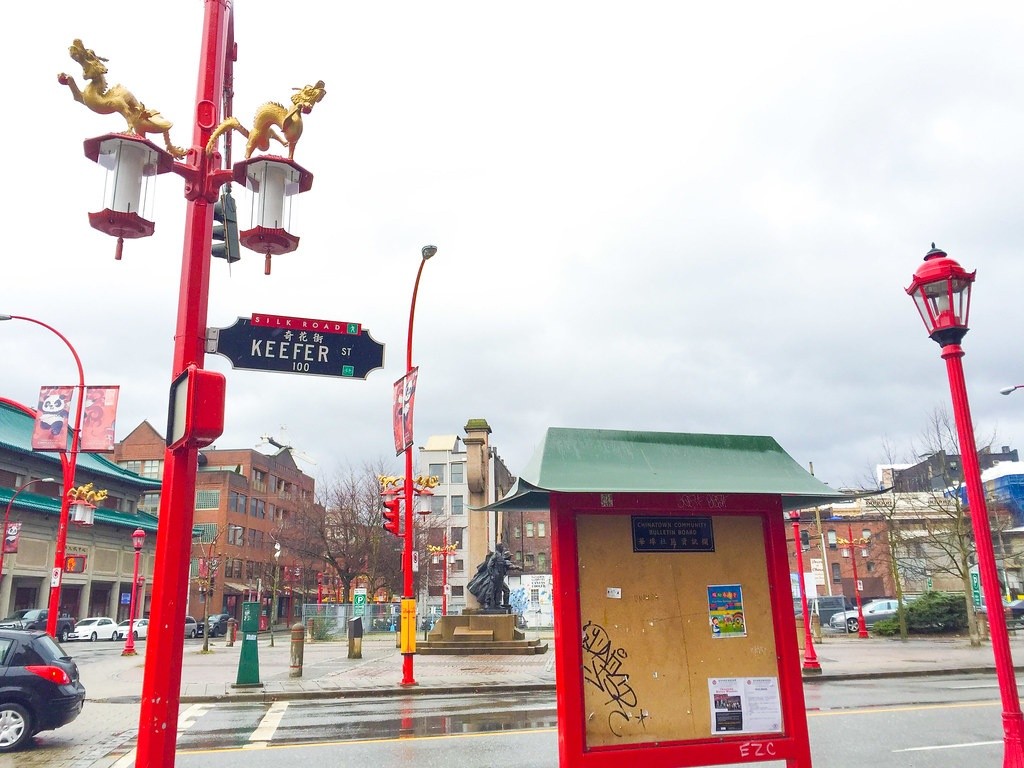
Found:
[67,617,120,642]
[1003,600,1024,625]
[831,598,909,631]
[116,619,151,638]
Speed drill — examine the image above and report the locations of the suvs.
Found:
[0,629,86,751]
[969,596,1011,616]
[795,595,855,627]
[183,615,198,639]
[196,613,231,637]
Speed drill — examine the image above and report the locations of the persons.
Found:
[484,542,523,610]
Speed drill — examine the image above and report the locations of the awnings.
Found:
[225,581,339,596]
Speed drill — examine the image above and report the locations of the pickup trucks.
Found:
[0,608,76,642]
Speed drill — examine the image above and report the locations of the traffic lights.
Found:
[379,499,401,539]
[64,554,86,574]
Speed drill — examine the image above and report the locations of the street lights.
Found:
[0,313,107,637]
[425,534,460,616]
[58,37,328,768]
[317,571,324,613]
[834,523,874,640]
[0,477,56,592]
[902,240,1024,768]
[400,243,440,687]
[121,526,148,656]
